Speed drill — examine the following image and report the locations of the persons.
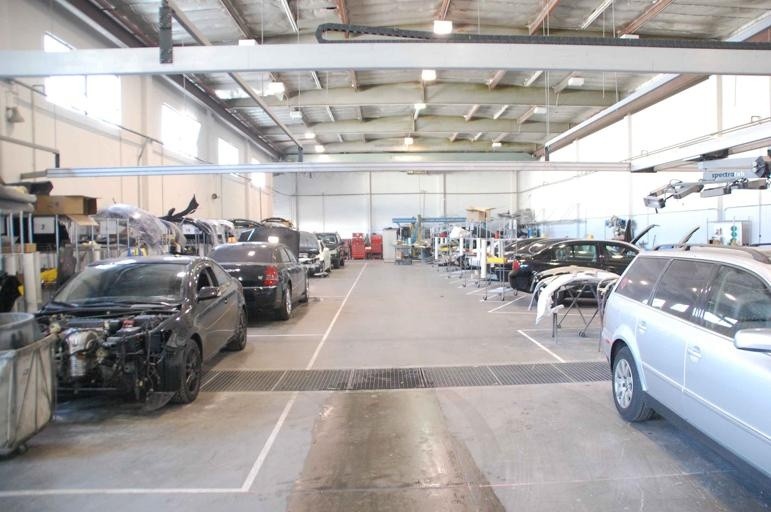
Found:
[89,231,105,264]
[0,269,26,313]
[72,236,92,274]
[175,238,182,255]
[459,225,470,247]
[169,238,176,255]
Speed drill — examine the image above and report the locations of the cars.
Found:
[458,235,651,305]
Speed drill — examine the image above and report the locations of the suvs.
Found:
[595,240,771,493]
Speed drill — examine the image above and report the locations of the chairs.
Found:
[734,292,771,324]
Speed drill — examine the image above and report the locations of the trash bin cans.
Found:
[0,311,57,460]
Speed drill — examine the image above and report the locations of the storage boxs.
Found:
[465,207,496,222]
[33,196,103,216]
